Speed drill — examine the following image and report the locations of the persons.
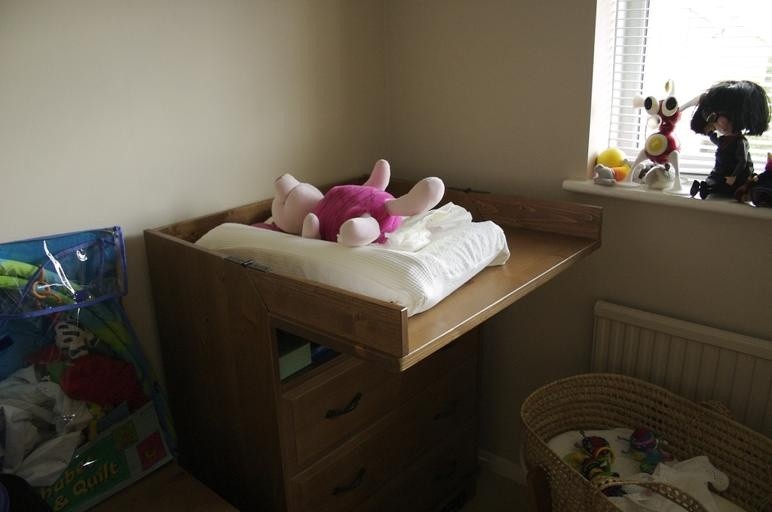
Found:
[691,82,772,201]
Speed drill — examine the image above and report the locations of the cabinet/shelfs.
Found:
[143,173,603,512]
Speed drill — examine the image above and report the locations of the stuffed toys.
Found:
[251,158,446,246]
[592,150,631,183]
[735,153,771,208]
[566,423,676,499]
[631,78,708,191]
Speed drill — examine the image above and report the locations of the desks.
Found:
[84,463,242,512]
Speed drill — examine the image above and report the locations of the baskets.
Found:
[521,374,772,512]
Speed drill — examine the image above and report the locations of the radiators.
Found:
[589,299,771,437]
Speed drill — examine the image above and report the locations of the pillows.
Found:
[195,211,512,317]
